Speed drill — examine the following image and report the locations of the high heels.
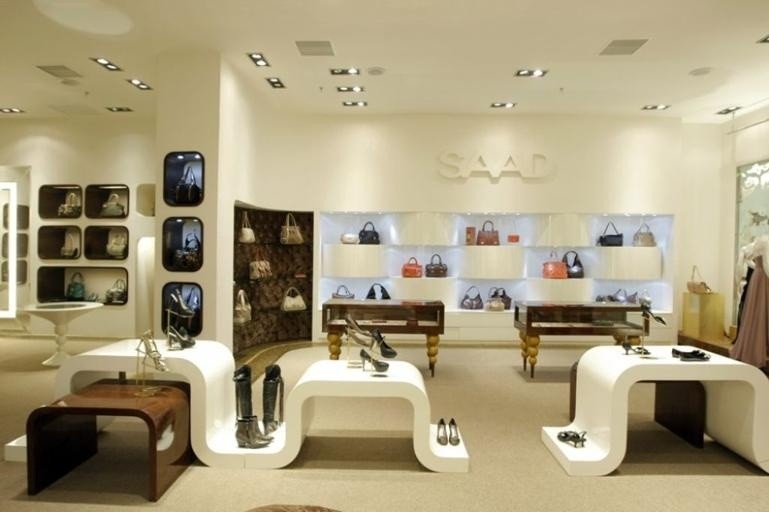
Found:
[360,350,388,373]
[137,331,168,373]
[165,324,195,348]
[623,342,650,355]
[369,328,397,358]
[642,304,667,326]
[558,431,586,448]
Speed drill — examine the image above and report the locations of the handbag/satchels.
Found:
[427,255,448,276]
[164,168,201,333]
[598,286,638,303]
[600,222,654,247]
[462,286,511,310]
[508,235,519,242]
[281,287,306,312]
[686,267,712,295]
[282,212,305,244]
[332,286,352,298]
[341,234,358,245]
[250,250,271,280]
[359,221,380,245]
[239,217,255,244]
[55,193,128,303]
[477,220,498,244]
[235,290,252,324]
[542,253,583,279]
[402,256,422,278]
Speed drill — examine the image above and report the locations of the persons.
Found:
[728,234,769,367]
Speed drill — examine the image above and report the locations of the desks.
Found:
[22,301,104,369]
[541,344,769,477]
[3,334,469,505]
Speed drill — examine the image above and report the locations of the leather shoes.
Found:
[343,314,370,335]
[340,328,369,347]
[437,418,448,445]
[449,418,459,445]
[672,348,709,361]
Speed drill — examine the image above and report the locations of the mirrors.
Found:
[0,165,31,312]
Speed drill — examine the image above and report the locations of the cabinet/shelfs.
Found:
[160,152,205,338]
[234,202,314,350]
[317,210,673,318]
[2,203,29,288]
[35,183,129,307]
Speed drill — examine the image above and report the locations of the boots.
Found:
[262,365,280,436]
[236,415,273,447]
[233,364,253,416]
